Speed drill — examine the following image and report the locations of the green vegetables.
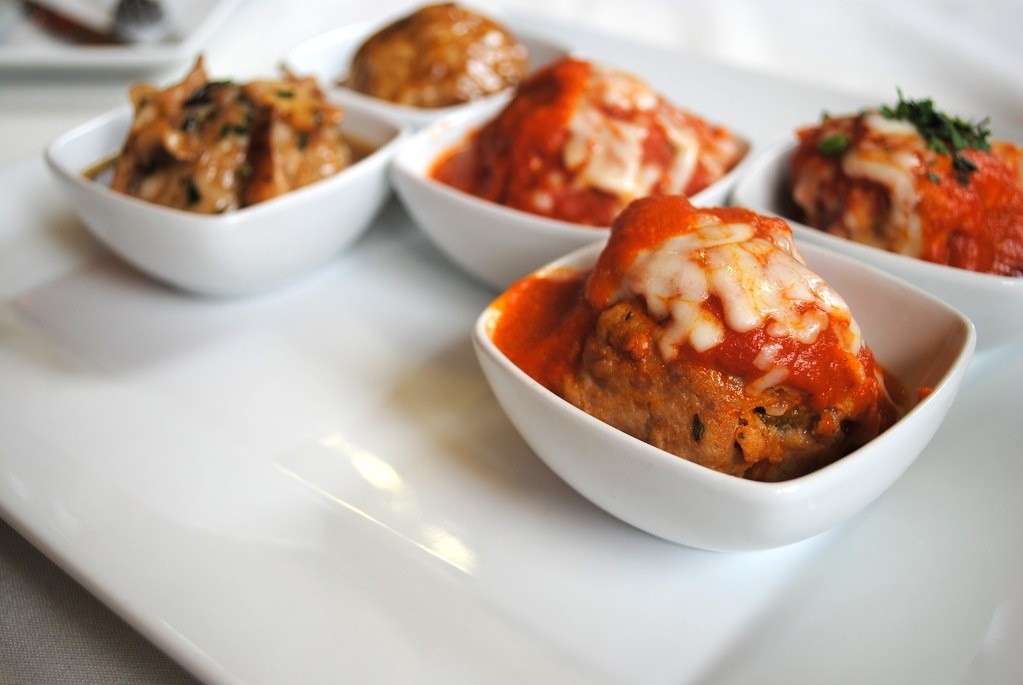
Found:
[178,78,314,206]
[813,85,993,186]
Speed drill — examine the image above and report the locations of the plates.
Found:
[2,1,1021,684]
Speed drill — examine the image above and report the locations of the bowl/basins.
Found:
[272,3,579,135]
[725,139,1023,352]
[470,236,979,553]
[384,89,766,292]
[44,80,413,297]
